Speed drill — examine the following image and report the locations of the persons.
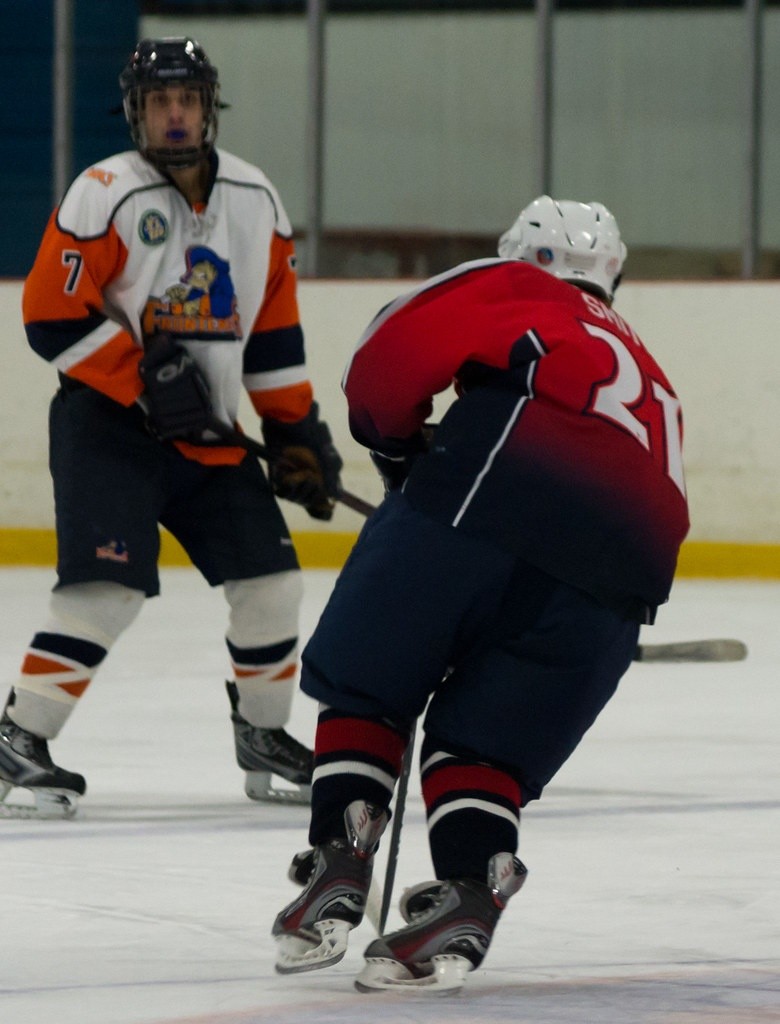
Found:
[276,197,692,995]
[1,38,341,817]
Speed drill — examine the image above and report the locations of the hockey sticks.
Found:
[207,414,747,666]
[287,717,417,933]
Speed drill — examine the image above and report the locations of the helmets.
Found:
[118,36,221,166]
[498,195,628,306]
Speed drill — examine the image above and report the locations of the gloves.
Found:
[140,333,214,445]
[260,404,344,521]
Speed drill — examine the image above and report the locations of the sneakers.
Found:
[272,799,392,976]
[0,685,87,822]
[353,851,527,998]
[225,680,315,801]
